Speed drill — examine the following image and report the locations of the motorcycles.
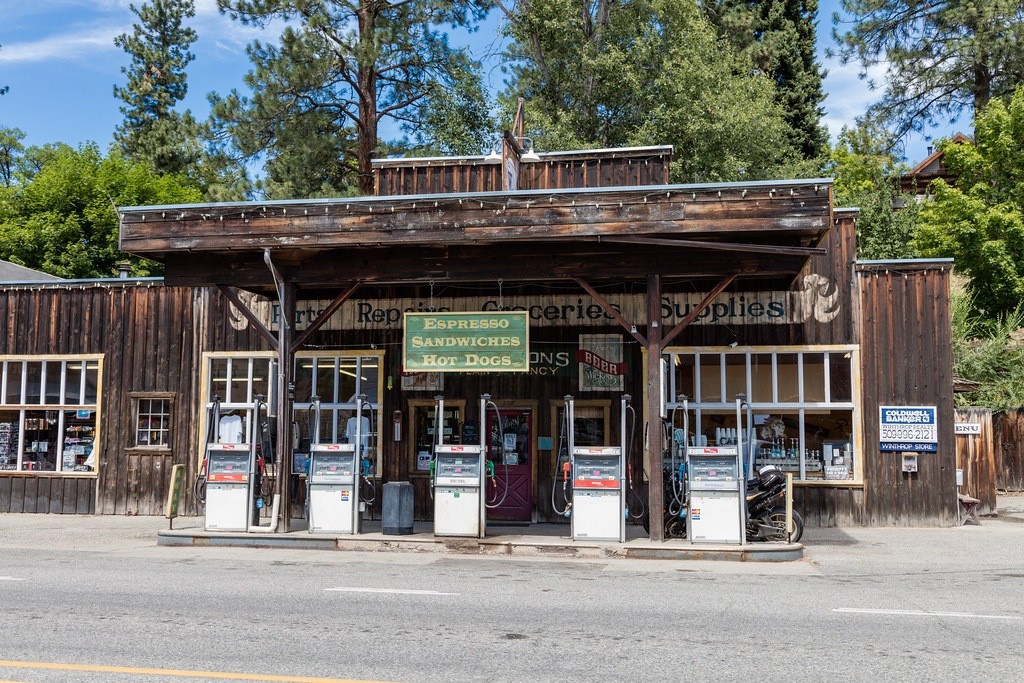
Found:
[642,467,804,546]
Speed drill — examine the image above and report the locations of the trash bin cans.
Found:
[382,481,414,535]
